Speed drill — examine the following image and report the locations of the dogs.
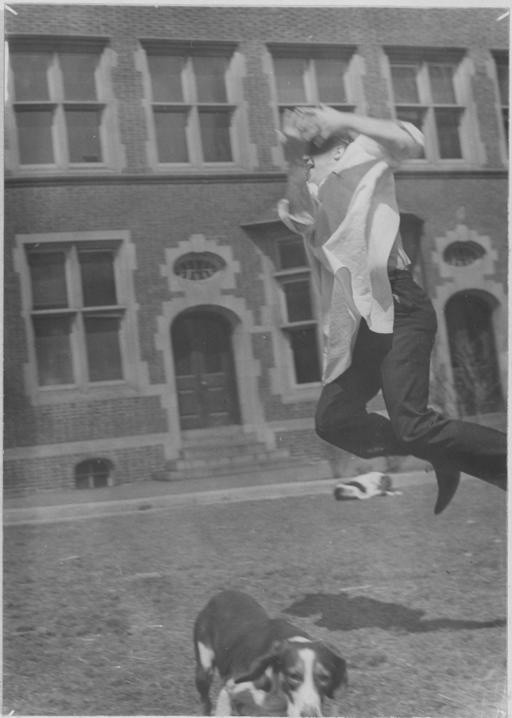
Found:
[334,470,401,502]
[194,590,347,717]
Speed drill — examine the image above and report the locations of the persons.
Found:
[271,102,507,515]
